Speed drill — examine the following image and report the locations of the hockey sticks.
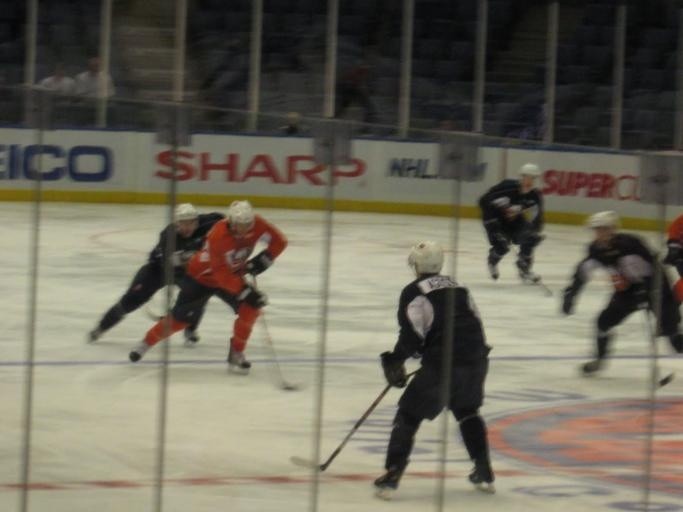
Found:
[291,381,393,473]
[146,310,164,321]
[254,275,308,393]
[644,306,675,387]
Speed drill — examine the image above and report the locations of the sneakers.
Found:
[488,261,500,280]
[519,269,541,281]
[185,333,201,343]
[374,473,400,489]
[583,361,602,372]
[468,464,494,483]
[228,351,251,369]
[130,341,151,362]
[88,325,104,341]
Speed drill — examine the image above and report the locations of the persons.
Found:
[373,240,495,490]
[561,208,682,373]
[75,58,117,99]
[479,164,545,283]
[89,203,226,343]
[35,59,75,94]
[129,199,288,369]
[661,213,683,361]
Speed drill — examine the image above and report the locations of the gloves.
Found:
[246,250,272,276]
[380,351,408,388]
[234,285,267,308]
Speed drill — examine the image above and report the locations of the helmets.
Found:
[406,241,444,277]
[520,164,541,193]
[174,204,201,237]
[229,201,257,239]
[587,212,622,246]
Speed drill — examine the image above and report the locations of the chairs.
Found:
[0,0,681,149]
